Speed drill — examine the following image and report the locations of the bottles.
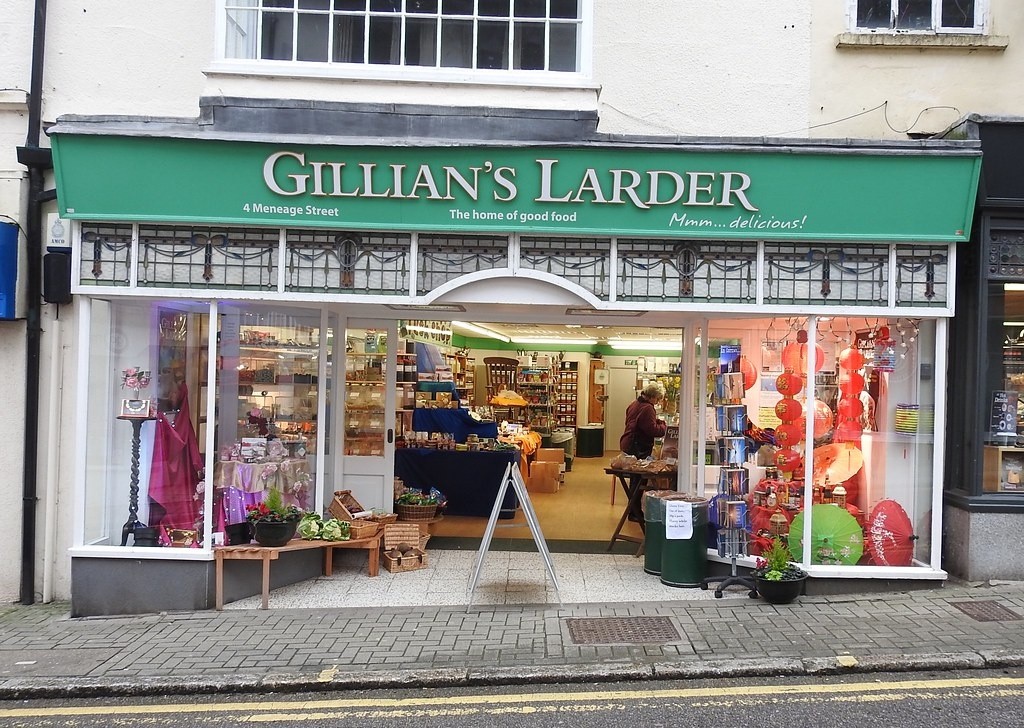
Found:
[821,473,832,504]
[767,487,777,507]
[812,478,821,505]
[777,476,786,503]
[308,386,318,415]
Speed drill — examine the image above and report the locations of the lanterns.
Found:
[717,357,757,390]
[837,420,863,449]
[797,330,808,343]
[878,326,889,340]
[773,447,800,478]
[782,341,824,374]
[840,398,864,418]
[840,373,864,394]
[774,421,801,446]
[775,369,802,396]
[775,397,802,421]
[840,347,863,370]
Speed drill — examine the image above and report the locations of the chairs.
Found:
[483,357,528,424]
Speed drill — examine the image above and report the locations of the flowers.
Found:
[661,376,681,400]
[754,534,792,581]
[245,483,303,526]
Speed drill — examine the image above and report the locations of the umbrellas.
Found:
[800,443,863,486]
[867,500,919,566]
[787,504,864,566]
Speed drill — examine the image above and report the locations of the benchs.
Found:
[210,528,384,611]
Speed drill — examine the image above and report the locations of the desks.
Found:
[395,448,521,519]
[214,459,310,545]
[604,466,678,558]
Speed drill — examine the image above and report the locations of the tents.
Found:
[791,399,833,438]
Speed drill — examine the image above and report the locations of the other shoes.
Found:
[628,512,645,523]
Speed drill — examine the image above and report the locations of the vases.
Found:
[225,522,253,546]
[253,522,300,547]
[667,398,676,416]
[755,569,808,605]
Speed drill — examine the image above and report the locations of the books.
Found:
[715,345,746,528]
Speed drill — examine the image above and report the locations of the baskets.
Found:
[329,489,431,573]
[394,500,437,521]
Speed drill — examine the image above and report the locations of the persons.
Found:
[619,381,667,522]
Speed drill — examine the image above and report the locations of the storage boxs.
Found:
[327,476,438,574]
[527,449,566,493]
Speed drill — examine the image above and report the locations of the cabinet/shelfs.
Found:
[518,362,578,436]
[984,444,1024,492]
[197,345,475,458]
